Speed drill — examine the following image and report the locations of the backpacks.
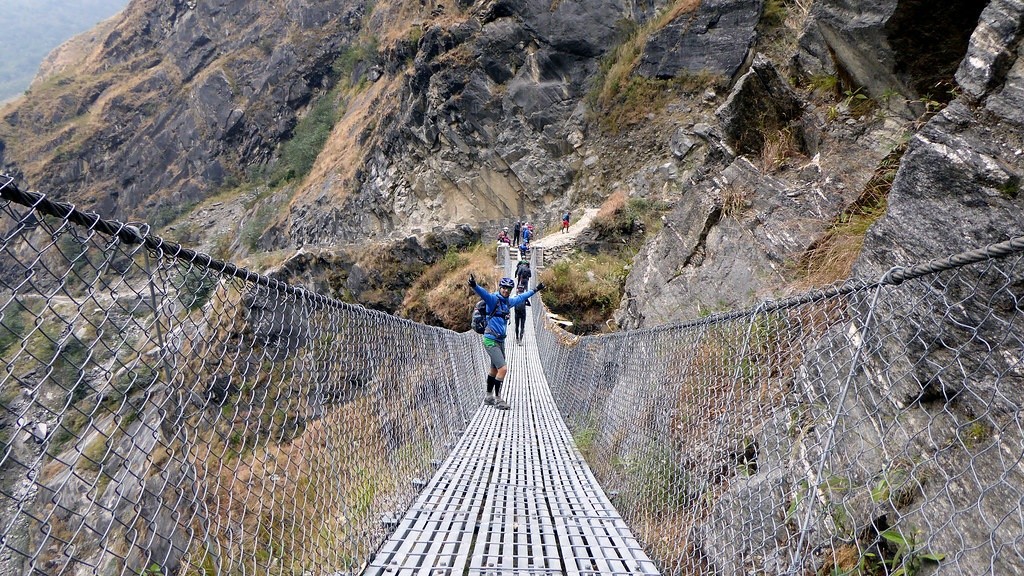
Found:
[516,293,525,310]
[521,268,530,279]
[472,297,509,334]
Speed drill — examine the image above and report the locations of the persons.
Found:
[561,212,572,234]
[467,273,545,410]
[498,220,534,346]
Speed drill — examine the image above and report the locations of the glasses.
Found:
[501,287,512,290]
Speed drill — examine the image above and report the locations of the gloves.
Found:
[468,273,476,288]
[537,283,545,290]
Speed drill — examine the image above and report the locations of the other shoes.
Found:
[494,399,510,410]
[516,340,524,346]
[483,392,494,405]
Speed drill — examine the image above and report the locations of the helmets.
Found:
[518,284,524,288]
[499,278,514,288]
